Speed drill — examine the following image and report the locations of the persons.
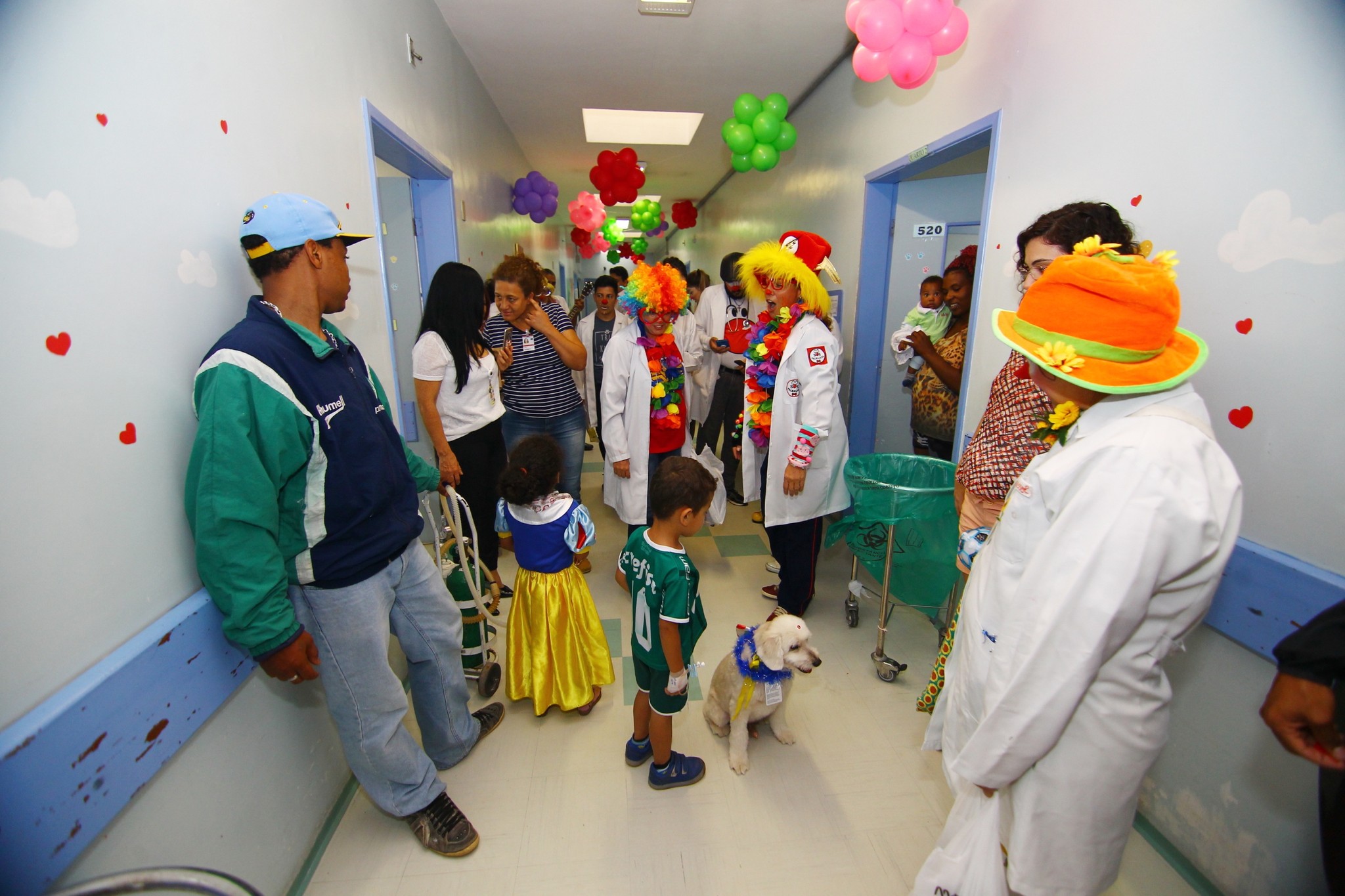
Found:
[1258,590,1345,895]
[911,243,978,460]
[627,455,721,789]
[890,276,953,386]
[187,194,510,860]
[917,199,1145,715]
[493,436,612,722]
[410,226,854,625]
[600,262,693,556]
[901,236,1245,894]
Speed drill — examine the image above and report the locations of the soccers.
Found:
[956,525,994,571]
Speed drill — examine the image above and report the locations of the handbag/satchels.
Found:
[911,779,1010,896]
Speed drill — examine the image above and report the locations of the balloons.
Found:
[569,193,699,266]
[721,92,797,172]
[838,0,968,90]
[512,171,561,224]
[589,143,648,206]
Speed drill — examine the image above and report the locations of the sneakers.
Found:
[762,585,779,599]
[765,560,781,573]
[648,750,706,790]
[766,606,794,622]
[625,738,653,767]
[404,791,479,856]
[471,702,506,742]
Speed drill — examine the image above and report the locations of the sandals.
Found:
[578,684,602,716]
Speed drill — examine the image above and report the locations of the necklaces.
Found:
[637,317,683,431]
[746,297,809,448]
[258,298,340,351]
[733,297,746,312]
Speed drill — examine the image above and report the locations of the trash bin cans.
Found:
[844,454,960,682]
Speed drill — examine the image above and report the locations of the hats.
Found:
[992,234,1209,394]
[754,230,832,285]
[239,192,374,260]
[720,252,746,282]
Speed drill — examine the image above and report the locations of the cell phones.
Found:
[501,326,513,358]
[715,340,729,348]
[734,360,745,367]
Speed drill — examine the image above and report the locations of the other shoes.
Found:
[576,557,592,574]
[486,601,499,616]
[499,585,513,598]
[584,443,594,451]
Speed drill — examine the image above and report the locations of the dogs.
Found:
[702,614,823,775]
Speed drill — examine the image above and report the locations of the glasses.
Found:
[760,276,797,290]
[1019,265,1047,280]
[637,308,679,324]
